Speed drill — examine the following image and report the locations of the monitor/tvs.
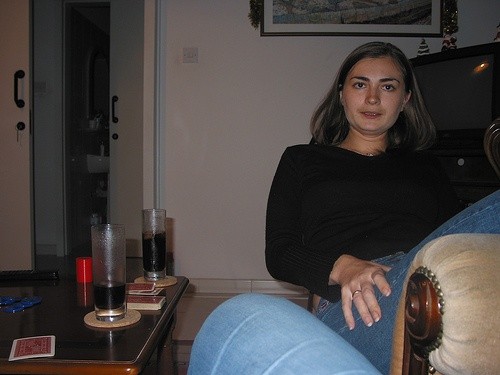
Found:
[404,41,500,141]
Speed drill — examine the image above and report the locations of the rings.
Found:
[352,290,362,298]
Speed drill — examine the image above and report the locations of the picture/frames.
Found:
[259,0,445,38]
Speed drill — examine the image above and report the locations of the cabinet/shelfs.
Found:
[427,148,500,208]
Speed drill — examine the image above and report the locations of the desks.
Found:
[0,262,188,375]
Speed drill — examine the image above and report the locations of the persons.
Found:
[186,293,383,375]
[266,42,500,375]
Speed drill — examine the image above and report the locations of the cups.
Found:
[141,208,167,281]
[75,256,92,283]
[91,223,127,322]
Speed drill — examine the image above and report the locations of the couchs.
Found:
[390,232,500,375]
